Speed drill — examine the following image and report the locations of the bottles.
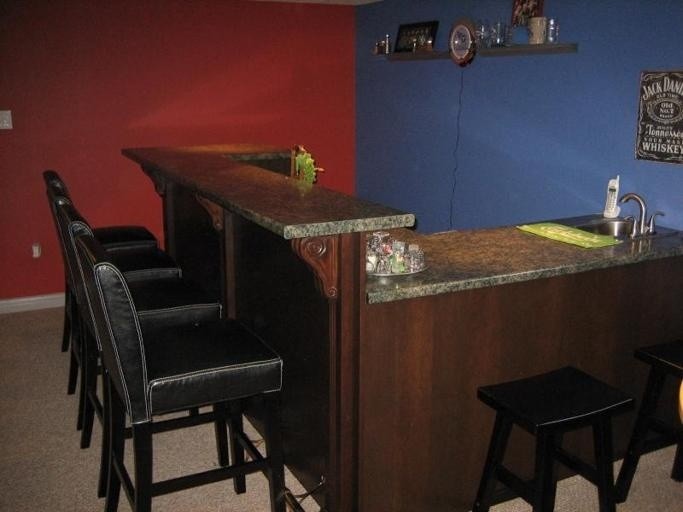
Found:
[528,16,560,45]
[384,33,391,54]
[366,232,426,274]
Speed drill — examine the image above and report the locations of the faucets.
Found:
[618,192,648,236]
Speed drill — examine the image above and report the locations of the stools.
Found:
[470,339,683,512]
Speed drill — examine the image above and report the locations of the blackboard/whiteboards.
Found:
[634,69,682,165]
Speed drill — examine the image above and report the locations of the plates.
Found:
[369,265,430,277]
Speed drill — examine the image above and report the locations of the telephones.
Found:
[603,175,620,218]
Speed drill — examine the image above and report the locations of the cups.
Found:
[476,19,509,48]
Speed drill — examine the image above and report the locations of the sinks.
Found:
[574,220,650,236]
